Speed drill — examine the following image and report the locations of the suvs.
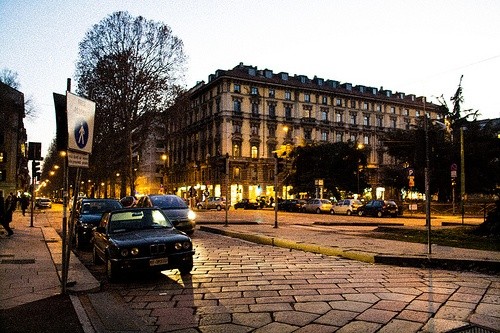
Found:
[196,196,231,211]
[357,200,398,218]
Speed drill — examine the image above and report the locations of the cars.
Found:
[35,196,64,209]
[277,198,300,211]
[329,199,364,216]
[234,198,260,209]
[302,198,332,213]
[67,199,125,250]
[90,207,196,281]
[137,193,196,237]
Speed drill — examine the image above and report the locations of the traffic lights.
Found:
[276,157,285,175]
[32,162,41,177]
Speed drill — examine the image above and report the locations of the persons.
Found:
[270,196,274,204]
[0,189,31,236]
